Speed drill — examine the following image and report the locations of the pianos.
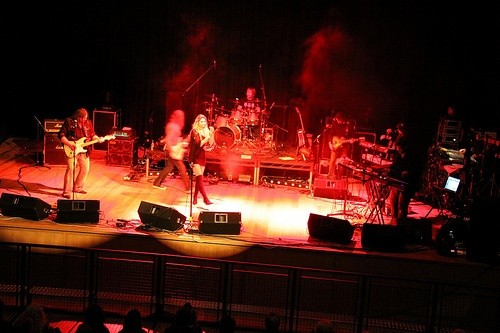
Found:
[339,140,407,224]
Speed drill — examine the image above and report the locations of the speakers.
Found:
[313,178,347,200]
[396,219,430,245]
[361,223,406,253]
[107,139,136,167]
[197,211,241,234]
[307,213,354,243]
[435,224,485,256]
[57,199,100,224]
[42,133,69,166]
[0,193,51,220]
[319,129,353,177]
[137,201,186,231]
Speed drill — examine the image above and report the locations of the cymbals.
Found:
[205,93,223,101]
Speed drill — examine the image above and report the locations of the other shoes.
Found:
[186,188,195,194]
[152,185,167,189]
[71,190,86,194]
[62,194,71,199]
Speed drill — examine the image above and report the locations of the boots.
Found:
[194,183,198,204]
[196,175,213,205]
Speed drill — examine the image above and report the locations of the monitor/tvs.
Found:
[444,175,462,193]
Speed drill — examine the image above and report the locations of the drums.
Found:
[246,112,259,126]
[230,110,244,125]
[214,123,242,150]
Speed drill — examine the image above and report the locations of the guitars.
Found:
[64,134,116,158]
[170,133,190,160]
[328,136,365,151]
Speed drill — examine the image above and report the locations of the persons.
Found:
[230,86,260,124]
[0,302,335,333]
[390,127,423,225]
[132,113,164,168]
[58,108,105,199]
[152,110,194,194]
[188,114,213,207]
[327,111,356,181]
